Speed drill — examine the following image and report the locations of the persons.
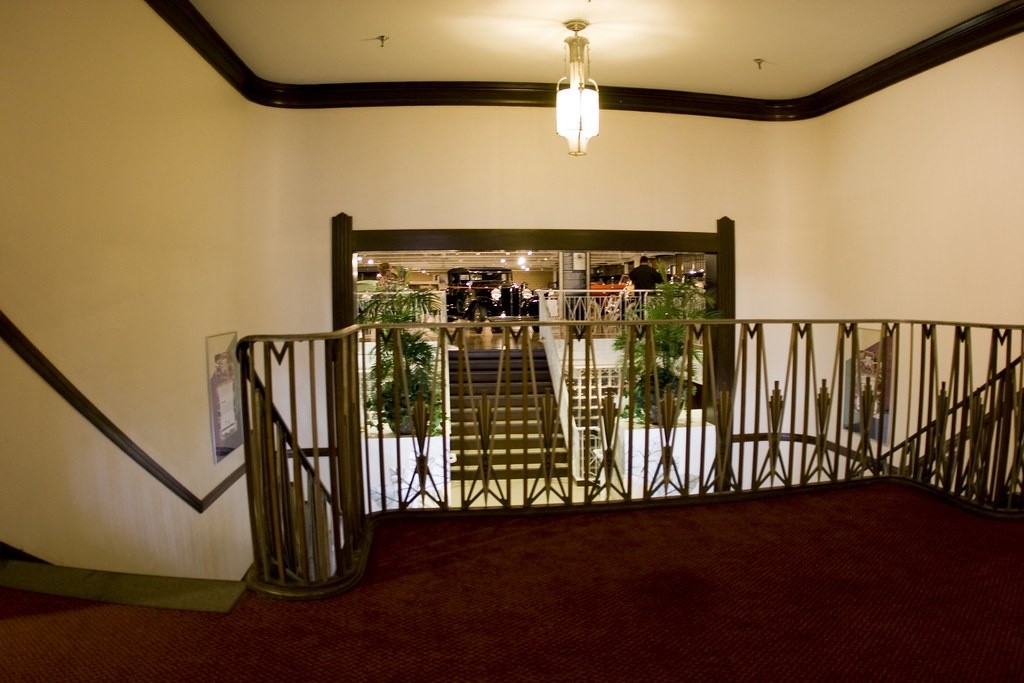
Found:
[630,256,662,321]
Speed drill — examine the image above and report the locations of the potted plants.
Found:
[354,264,451,436]
[611,261,727,426]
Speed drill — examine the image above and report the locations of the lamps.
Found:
[555,19,601,156]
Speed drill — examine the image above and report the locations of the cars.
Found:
[520,273,633,322]
[444,265,531,335]
